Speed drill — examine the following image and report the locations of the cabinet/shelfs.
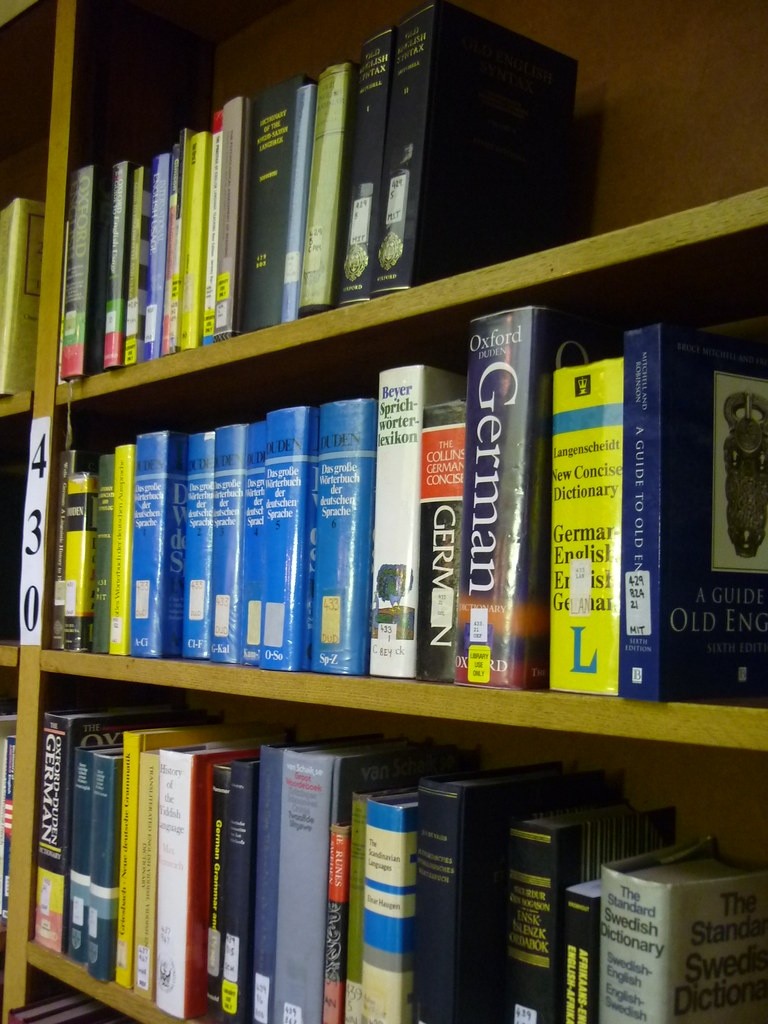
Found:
[3,0,765,1021]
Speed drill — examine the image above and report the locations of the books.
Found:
[0,0,767,1022]
